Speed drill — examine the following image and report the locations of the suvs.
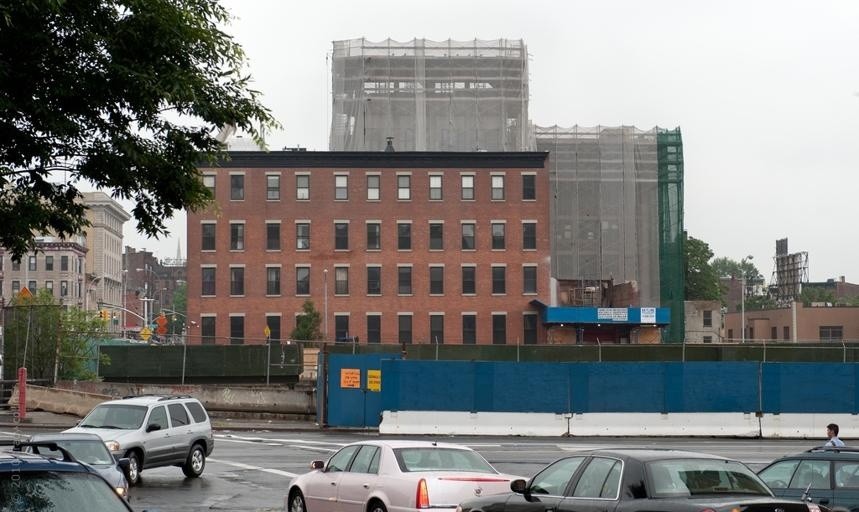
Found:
[61,394,215,488]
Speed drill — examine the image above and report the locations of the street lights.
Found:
[136,267,163,315]
[322,268,328,336]
[85,274,113,327]
[742,255,753,345]
[149,288,167,325]
[108,269,128,303]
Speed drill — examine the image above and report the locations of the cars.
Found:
[283,440,532,512]
[755,446,859,512]
[0,440,131,512]
[24,432,131,503]
[457,448,833,512]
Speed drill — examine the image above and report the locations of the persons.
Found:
[824,423,845,447]
